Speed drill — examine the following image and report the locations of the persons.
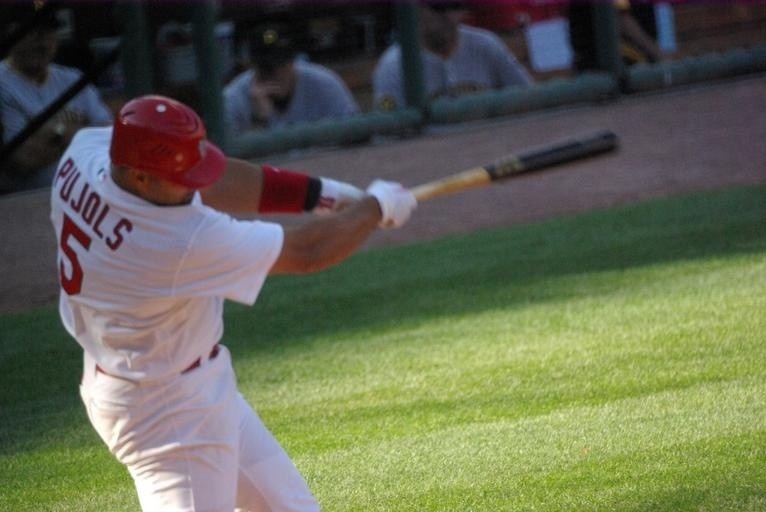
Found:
[48,95,418,512]
[1,2,675,180]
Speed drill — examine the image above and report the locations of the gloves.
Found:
[311,177,363,221]
[368,181,416,229]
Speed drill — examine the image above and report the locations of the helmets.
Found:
[108,95,227,187]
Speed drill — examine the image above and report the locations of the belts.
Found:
[187,345,220,372]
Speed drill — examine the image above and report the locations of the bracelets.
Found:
[54,121,65,148]
[306,175,322,212]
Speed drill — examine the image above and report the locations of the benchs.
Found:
[103,1,766,171]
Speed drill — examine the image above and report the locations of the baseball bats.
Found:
[411,127,619,199]
